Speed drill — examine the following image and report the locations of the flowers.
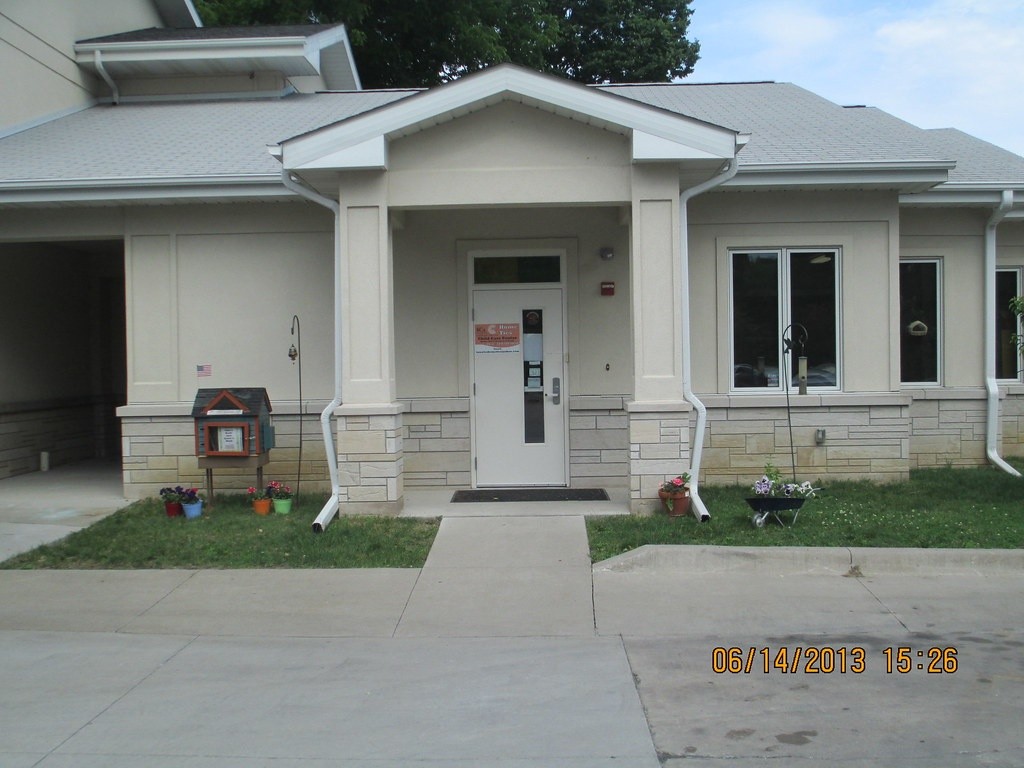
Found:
[159,486,200,506]
[247,480,293,501]
[751,462,812,497]
[661,472,691,511]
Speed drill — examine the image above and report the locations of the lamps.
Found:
[798,342,807,395]
[288,344,297,365]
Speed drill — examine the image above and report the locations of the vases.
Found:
[273,499,293,515]
[181,500,203,519]
[165,503,183,518]
[253,500,271,515]
[658,487,690,517]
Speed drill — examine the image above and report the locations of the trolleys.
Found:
[745,488,825,532]
